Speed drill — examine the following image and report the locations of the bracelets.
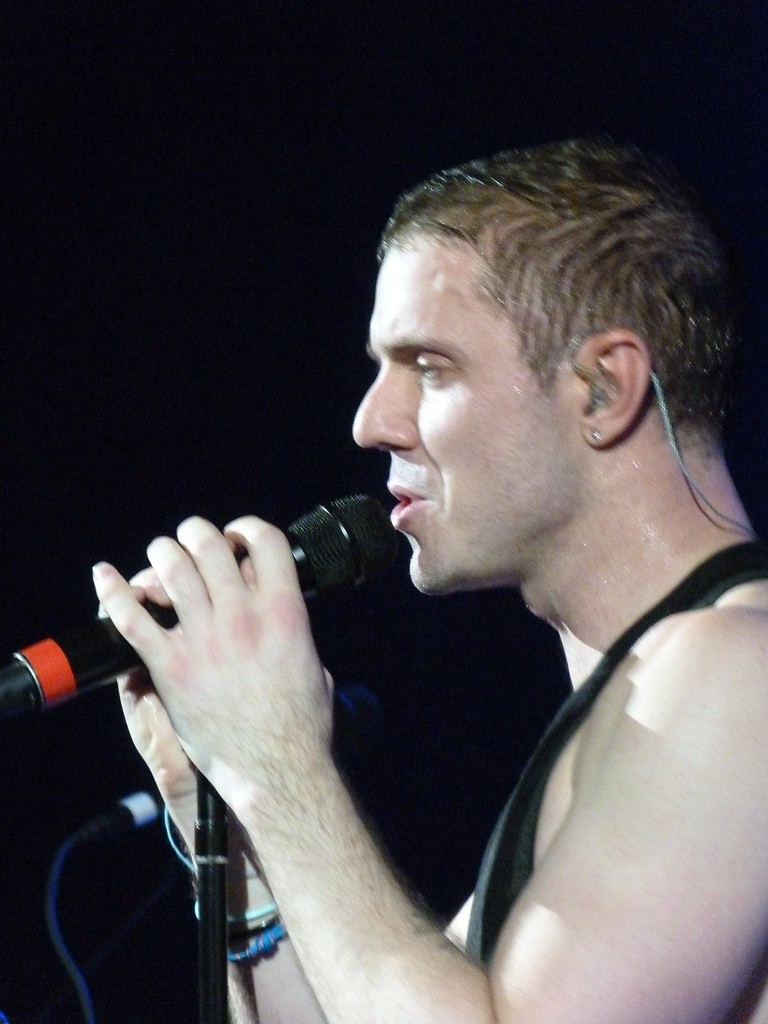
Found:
[191,896,287,963]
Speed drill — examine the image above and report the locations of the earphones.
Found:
[585,388,608,415]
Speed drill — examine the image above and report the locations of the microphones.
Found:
[0,495,395,725]
[76,791,167,845]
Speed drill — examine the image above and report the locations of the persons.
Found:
[92,134,768,1024]
